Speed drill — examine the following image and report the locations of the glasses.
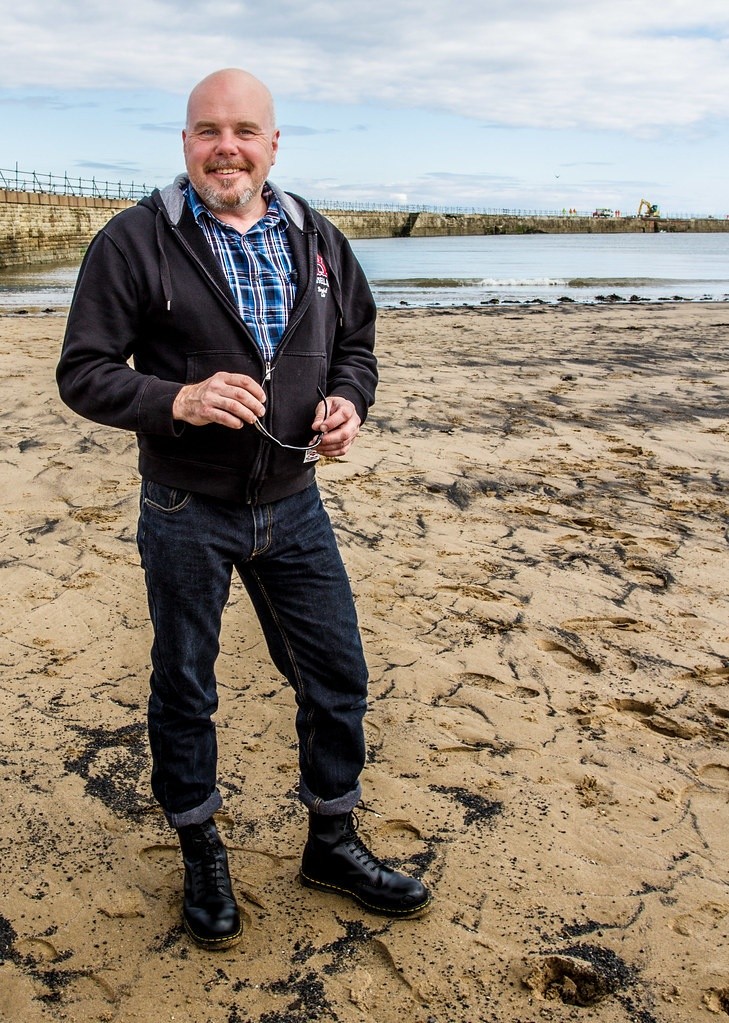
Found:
[252,368,329,450]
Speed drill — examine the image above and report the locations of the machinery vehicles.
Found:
[638,199,660,221]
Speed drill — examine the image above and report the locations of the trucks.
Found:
[596,208,614,217]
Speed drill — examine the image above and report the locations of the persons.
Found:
[56,66,431,953]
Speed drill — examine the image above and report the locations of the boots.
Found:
[178,817,245,948]
[296,809,433,918]
